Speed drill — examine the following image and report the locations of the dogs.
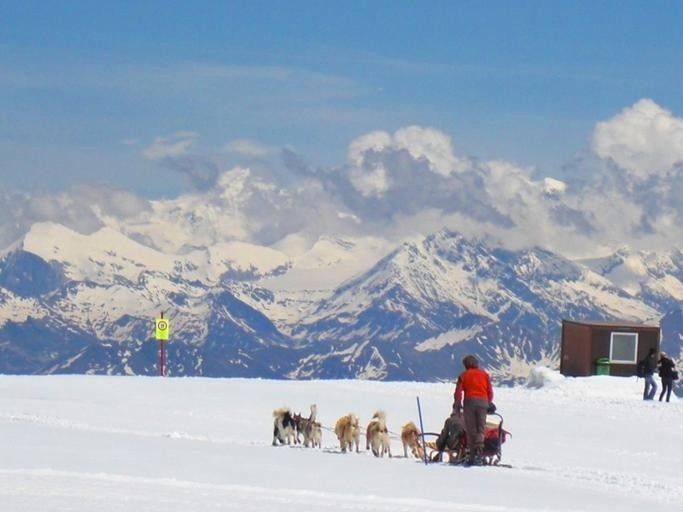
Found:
[272,403,323,449]
[334,414,360,452]
[401,421,425,459]
[366,411,393,458]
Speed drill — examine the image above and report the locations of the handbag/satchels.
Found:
[636,360,646,378]
[671,366,678,380]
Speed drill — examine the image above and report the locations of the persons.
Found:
[642,347,657,401]
[434,401,462,461]
[453,354,494,468]
[658,350,675,403]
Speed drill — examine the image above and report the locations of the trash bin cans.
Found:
[595,357,609,376]
